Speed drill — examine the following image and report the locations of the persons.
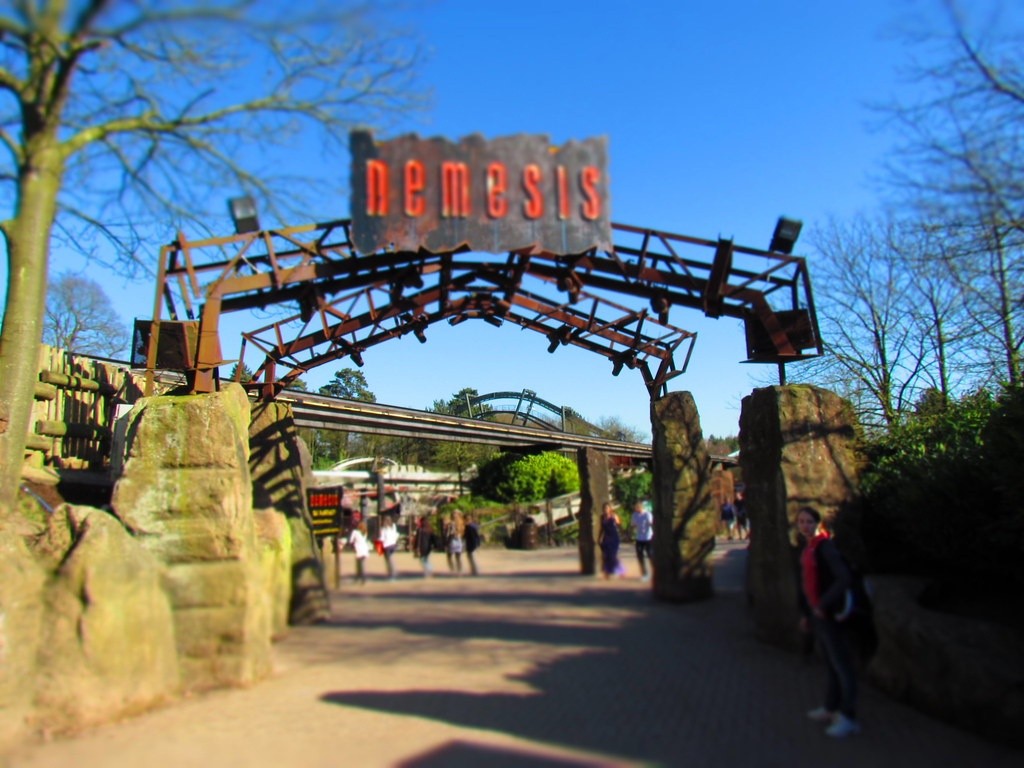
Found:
[413,512,435,578]
[349,521,370,586]
[378,515,401,580]
[720,491,750,541]
[793,506,860,738]
[596,500,654,581]
[443,510,482,576]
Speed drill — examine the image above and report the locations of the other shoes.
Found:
[640,574,649,582]
[727,536,733,540]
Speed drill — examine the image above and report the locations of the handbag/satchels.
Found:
[414,547,420,557]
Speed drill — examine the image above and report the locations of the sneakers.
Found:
[806,707,833,721]
[824,712,861,738]
